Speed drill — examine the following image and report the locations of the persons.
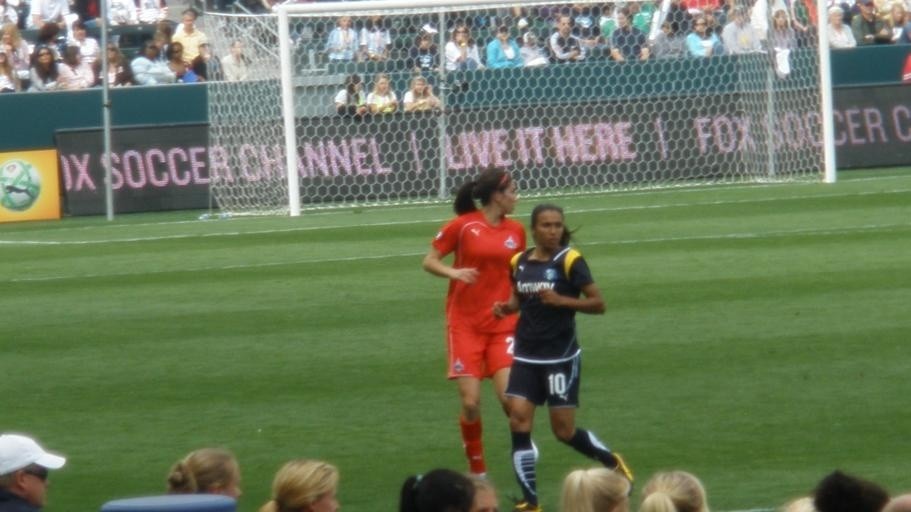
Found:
[259,457,341,511]
[492,202,635,511]
[2,1,251,93]
[399,468,478,511]
[262,0,818,118]
[558,466,632,511]
[424,167,529,504]
[0,433,66,512]
[640,469,709,511]
[824,1,911,49]
[812,468,892,511]
[166,447,244,504]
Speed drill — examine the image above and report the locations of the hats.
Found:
[420,22,438,35]
[827,6,844,15]
[858,0,875,6]
[0,432,67,476]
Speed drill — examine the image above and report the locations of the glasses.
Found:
[25,466,49,482]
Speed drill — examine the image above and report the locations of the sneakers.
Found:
[609,451,635,496]
[513,500,543,512]
